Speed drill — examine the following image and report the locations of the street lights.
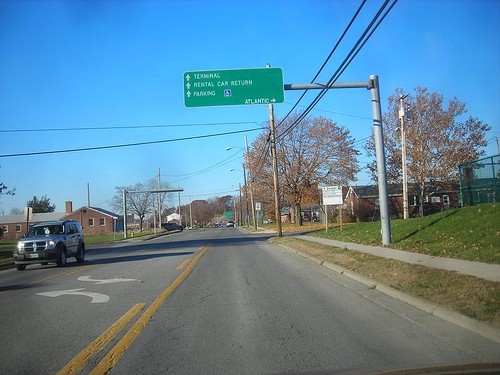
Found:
[226,135,257,230]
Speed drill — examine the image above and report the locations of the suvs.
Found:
[160,222,185,232]
[13,219,86,270]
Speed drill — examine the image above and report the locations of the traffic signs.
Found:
[183,67,284,108]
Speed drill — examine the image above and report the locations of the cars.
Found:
[226,221,235,228]
